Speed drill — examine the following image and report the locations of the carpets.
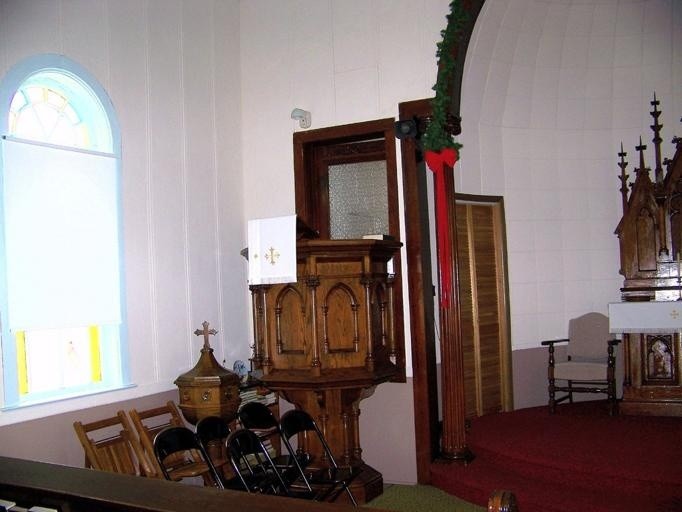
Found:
[360,485,489,511]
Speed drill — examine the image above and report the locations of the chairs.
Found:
[541,312,622,416]
[73,400,359,505]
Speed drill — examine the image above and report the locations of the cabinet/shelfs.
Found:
[174,370,278,488]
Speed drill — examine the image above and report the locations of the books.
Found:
[235,388,278,471]
